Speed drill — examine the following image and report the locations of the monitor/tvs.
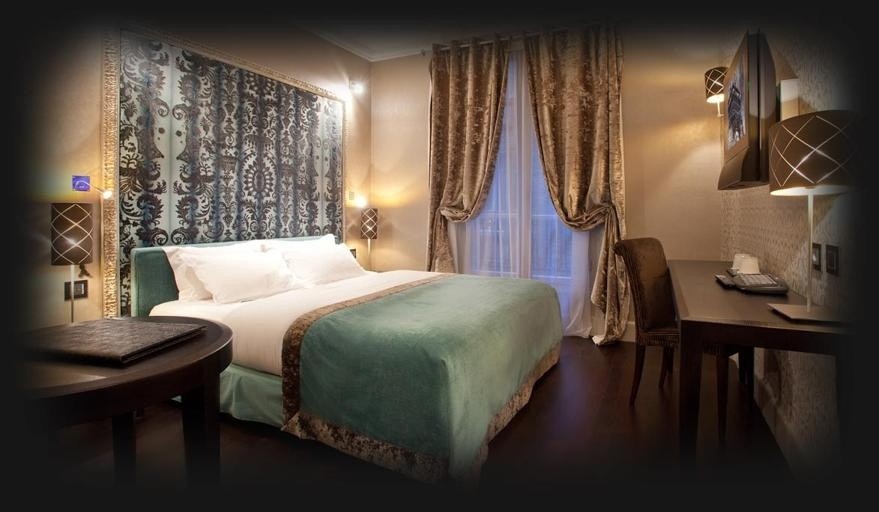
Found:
[718,27,777,190]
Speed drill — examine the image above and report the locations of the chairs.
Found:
[614,237,754,448]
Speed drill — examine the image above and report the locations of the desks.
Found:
[12,316,235,497]
[669,260,854,470]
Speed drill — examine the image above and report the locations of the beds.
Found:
[130,234,564,486]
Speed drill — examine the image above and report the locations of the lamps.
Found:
[360,209,379,269]
[703,66,728,116]
[52,203,95,327]
[767,108,856,325]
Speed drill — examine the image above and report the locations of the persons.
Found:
[726,81,742,112]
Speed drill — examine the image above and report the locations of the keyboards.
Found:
[733,272,788,295]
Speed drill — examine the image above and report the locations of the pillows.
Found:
[161,234,365,302]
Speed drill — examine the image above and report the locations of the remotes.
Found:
[715,274,736,288]
